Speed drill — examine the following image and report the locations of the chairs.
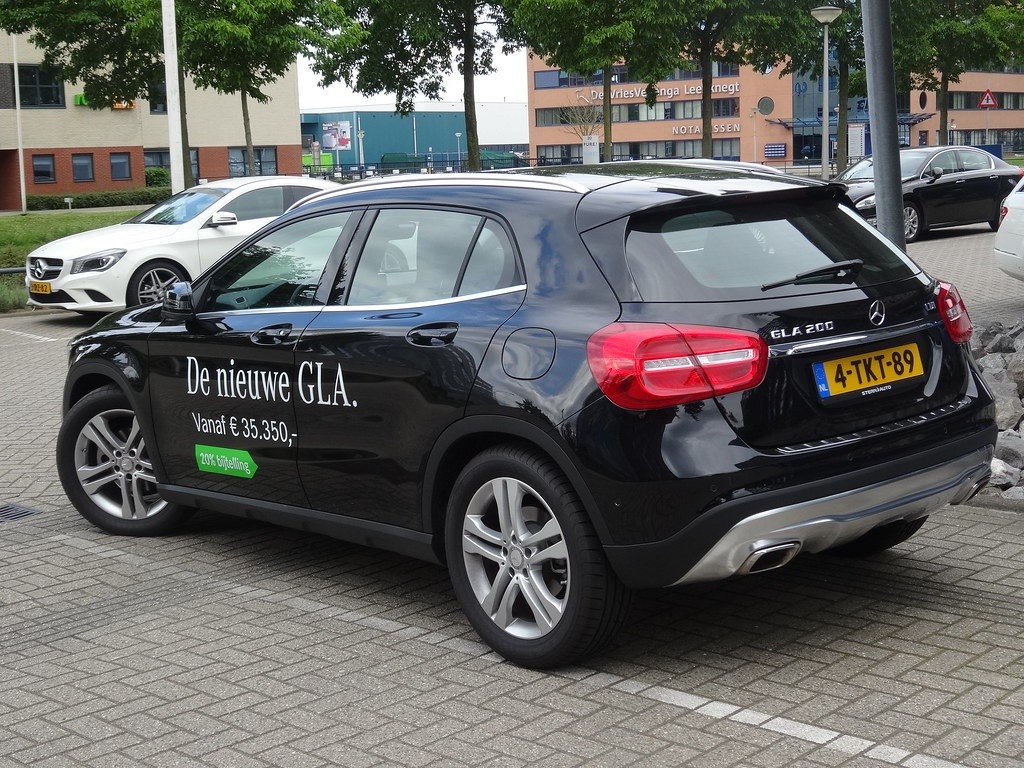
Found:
[964,156,981,171]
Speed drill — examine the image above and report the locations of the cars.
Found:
[824,147,1024,243]
[55,158,999,668]
[994,176,1024,282]
[23,176,493,319]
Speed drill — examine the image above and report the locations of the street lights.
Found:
[834,106,840,128]
[811,4,845,180]
[454,133,463,172]
